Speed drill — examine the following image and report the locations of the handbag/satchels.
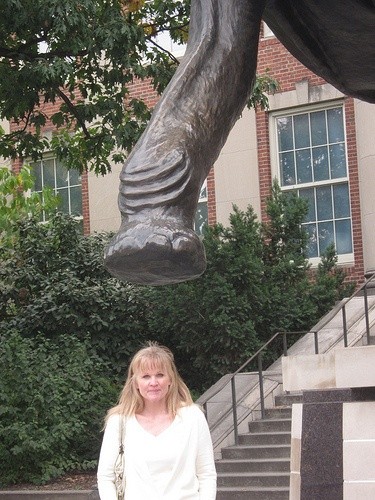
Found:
[113,415,126,500]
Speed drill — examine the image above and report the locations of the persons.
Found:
[97,341,217,500]
